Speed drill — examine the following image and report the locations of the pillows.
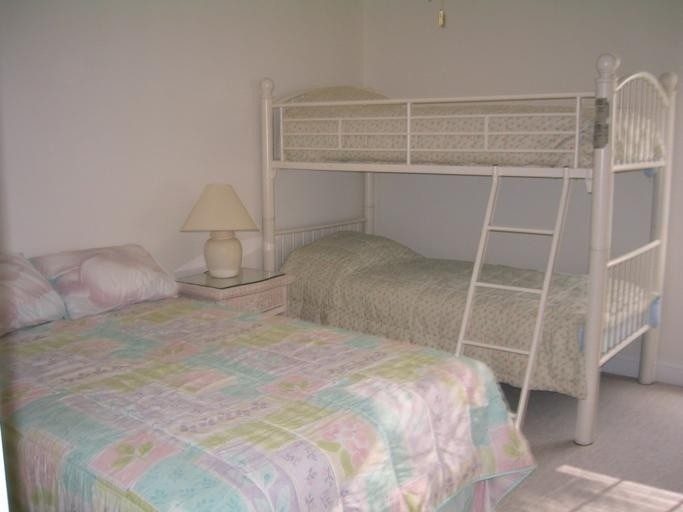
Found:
[0,252,66,337]
[27,244,178,320]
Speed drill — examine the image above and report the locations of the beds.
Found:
[0,296,495,512]
[260,53,678,446]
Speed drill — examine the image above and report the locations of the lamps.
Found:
[181,181,259,279]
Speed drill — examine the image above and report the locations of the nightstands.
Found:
[175,267,294,318]
